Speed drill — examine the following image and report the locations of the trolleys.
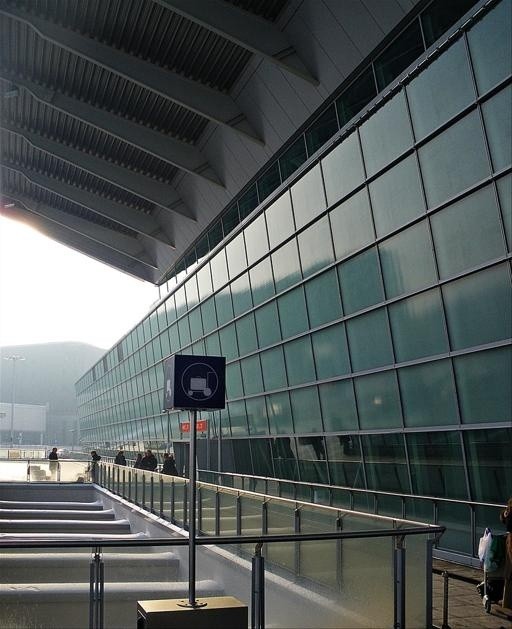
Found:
[476,526,510,613]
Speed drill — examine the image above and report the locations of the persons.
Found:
[49,448,58,481]
[114,450,179,476]
[89,450,101,483]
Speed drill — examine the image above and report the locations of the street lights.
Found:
[4,354,26,448]
